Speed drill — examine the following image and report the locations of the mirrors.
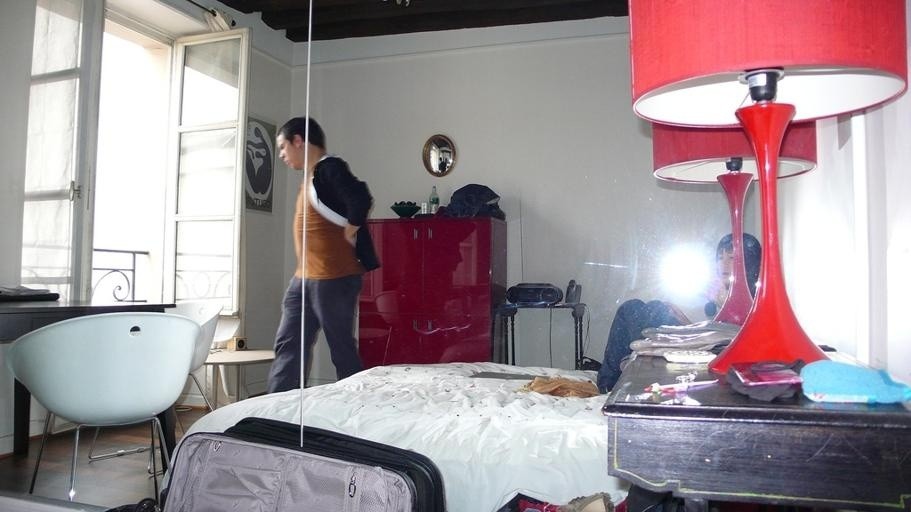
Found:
[422,134,456,177]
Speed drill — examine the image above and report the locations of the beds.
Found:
[210,362,632,512]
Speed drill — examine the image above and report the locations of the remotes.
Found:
[664,350,717,363]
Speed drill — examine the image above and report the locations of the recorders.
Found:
[507,283,563,304]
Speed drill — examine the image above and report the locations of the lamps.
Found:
[652,118,817,326]
[629,0,908,378]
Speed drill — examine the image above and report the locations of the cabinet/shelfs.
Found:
[358,215,508,371]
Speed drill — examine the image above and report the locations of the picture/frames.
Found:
[246,112,280,216]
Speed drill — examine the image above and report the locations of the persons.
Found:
[596,232,761,395]
[268,117,380,393]
[438,156,448,172]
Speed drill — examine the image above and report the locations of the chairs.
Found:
[88,298,224,474]
[6,312,200,511]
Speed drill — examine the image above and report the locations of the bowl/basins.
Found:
[390,206,421,218]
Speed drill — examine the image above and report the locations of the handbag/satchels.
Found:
[727,357,803,402]
[102,416,445,512]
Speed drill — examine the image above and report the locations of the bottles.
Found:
[429,186,439,215]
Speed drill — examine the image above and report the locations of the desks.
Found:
[204,348,276,411]
[602,339,911,512]
[0,298,177,492]
[495,302,585,370]
